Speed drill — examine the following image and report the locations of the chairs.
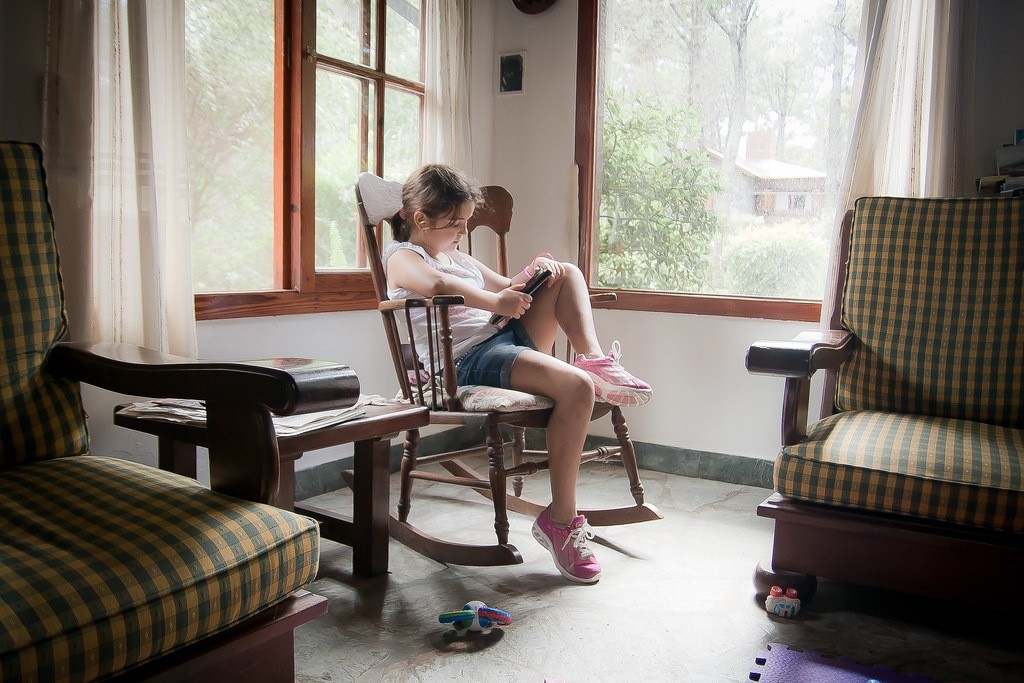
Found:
[342,172,665,567]
[0,137,360,683]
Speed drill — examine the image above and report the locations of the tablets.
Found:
[489,270,551,325]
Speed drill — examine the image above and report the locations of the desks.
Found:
[113,397,430,575]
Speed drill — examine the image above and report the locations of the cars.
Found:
[764,585,802,619]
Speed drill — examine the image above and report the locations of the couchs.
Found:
[744,196,1024,610]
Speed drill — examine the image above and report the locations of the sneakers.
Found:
[574,340,653,407]
[532,502,603,583]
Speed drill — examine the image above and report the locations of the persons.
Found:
[385,162,653,586]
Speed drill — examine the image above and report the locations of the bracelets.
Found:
[532,252,555,271]
[524,265,533,280]
